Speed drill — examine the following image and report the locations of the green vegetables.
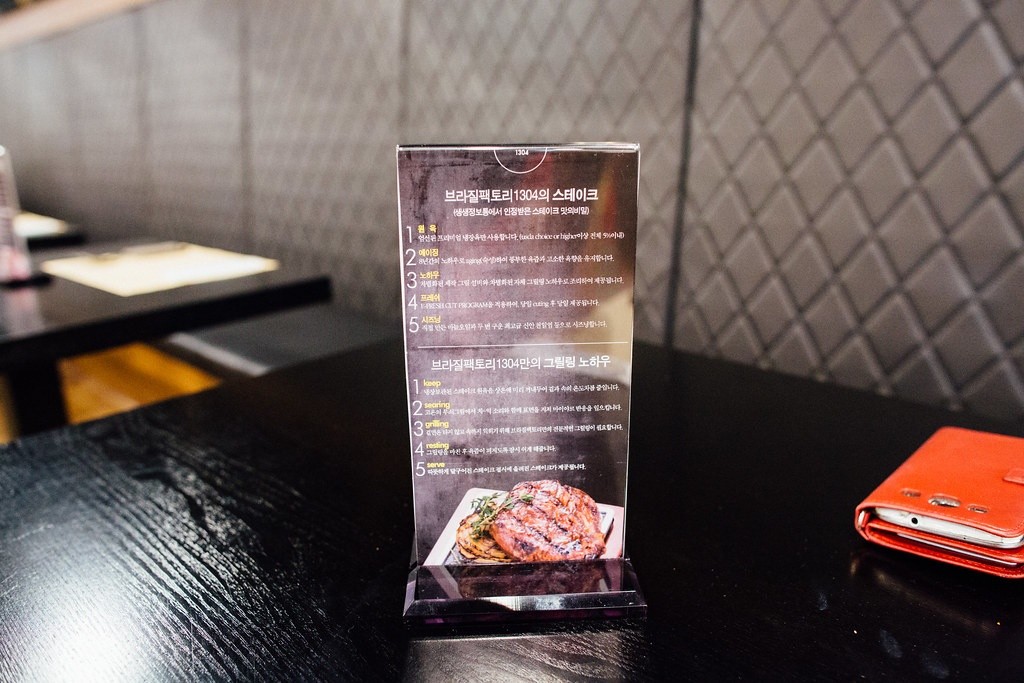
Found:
[469,490,530,535]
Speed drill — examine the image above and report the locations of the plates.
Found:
[422,487,625,564]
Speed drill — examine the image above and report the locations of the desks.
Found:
[0,209,334,440]
[1,338,1024,683]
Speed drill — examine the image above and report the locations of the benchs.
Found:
[160,304,403,379]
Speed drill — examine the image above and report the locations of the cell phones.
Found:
[875,427,1024,549]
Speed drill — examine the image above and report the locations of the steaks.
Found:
[491,481,606,562]
